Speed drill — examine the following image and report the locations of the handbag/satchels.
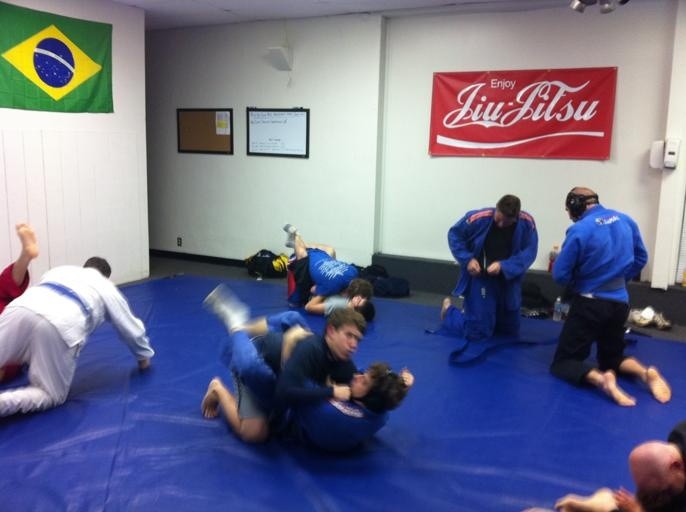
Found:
[247,250,289,279]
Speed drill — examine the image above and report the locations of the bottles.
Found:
[553,298,562,321]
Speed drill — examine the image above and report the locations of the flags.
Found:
[0,0,116,114]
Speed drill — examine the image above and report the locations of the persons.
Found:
[0,222,40,384]
[0,256,155,417]
[283,223,375,321]
[199,307,414,443]
[550,186,672,407]
[204,287,405,456]
[441,194,538,341]
[287,247,373,311]
[553,419,686,512]
